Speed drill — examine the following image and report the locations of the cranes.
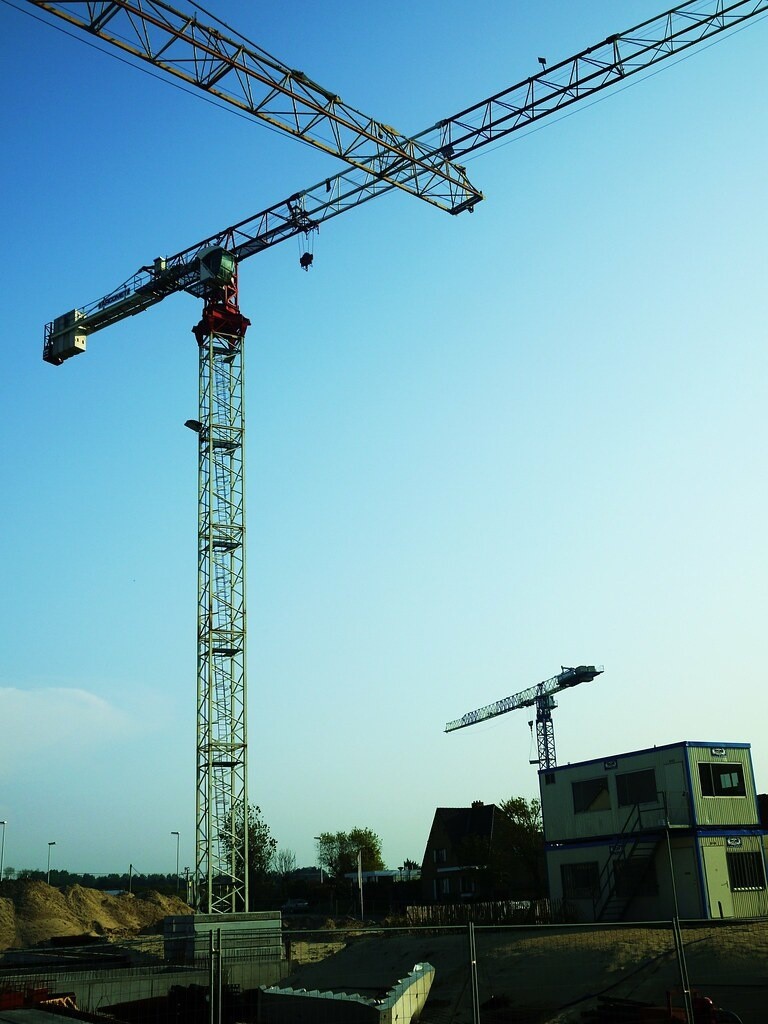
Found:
[0,0,768,918]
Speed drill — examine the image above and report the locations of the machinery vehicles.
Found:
[585,988,744,1024]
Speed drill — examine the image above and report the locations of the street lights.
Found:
[48,842,55,883]
[663,822,695,1024]
[0,820,8,881]
[169,831,179,893]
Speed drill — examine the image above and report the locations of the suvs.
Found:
[280,899,309,915]
